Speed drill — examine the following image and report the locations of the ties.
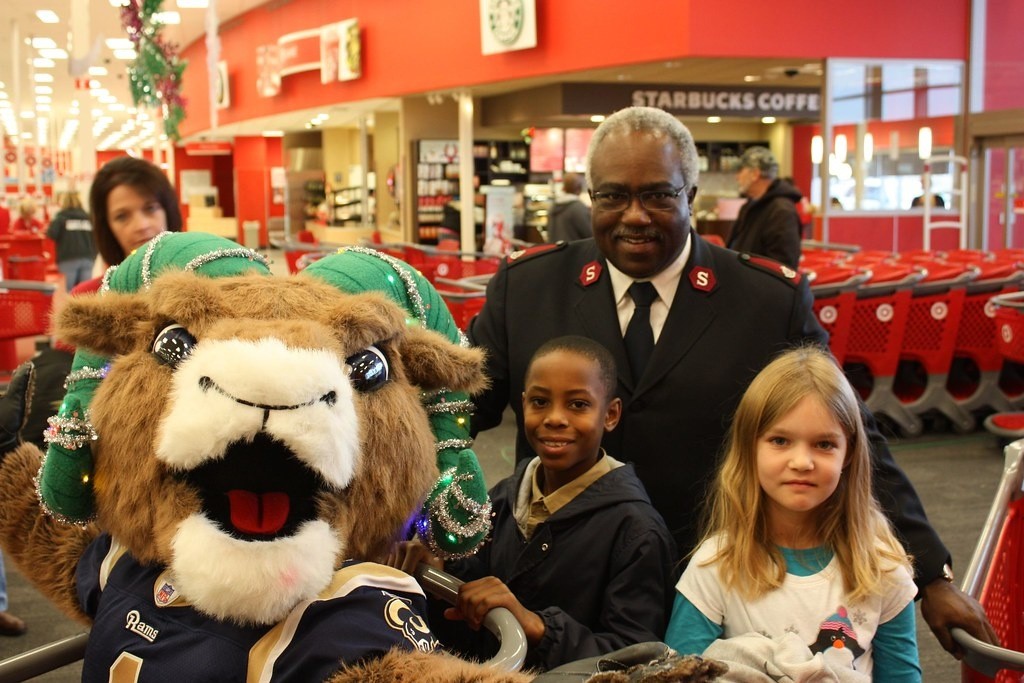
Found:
[622,280,659,389]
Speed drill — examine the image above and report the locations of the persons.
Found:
[467,105,1006,676]
[664,348,926,683]
[55,157,183,350]
[724,147,802,272]
[46,192,100,292]
[912,179,944,208]
[0,192,10,234]
[547,173,593,244]
[12,200,44,233]
[382,336,675,672]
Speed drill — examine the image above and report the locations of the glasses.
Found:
[592,184,686,212]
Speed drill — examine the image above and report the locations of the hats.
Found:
[734,147,780,180]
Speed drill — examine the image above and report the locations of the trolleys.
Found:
[0,229,58,343]
[285,231,1024,683]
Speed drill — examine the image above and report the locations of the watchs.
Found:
[933,563,954,584]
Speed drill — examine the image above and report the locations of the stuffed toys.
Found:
[0,231,534,683]
